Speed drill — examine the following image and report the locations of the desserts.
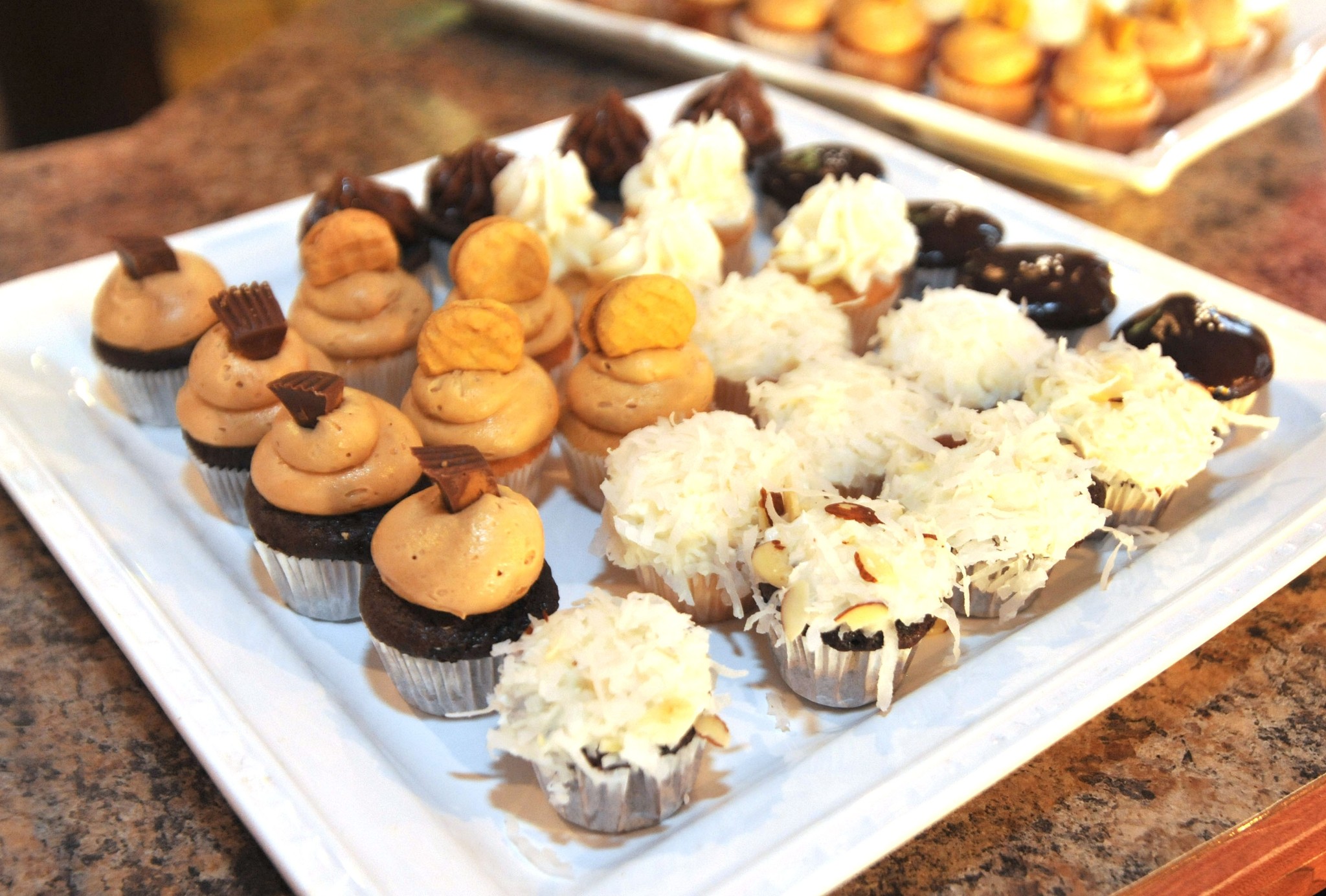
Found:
[729,1,1288,153]
[92,64,1274,831]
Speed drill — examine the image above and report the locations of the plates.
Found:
[474,0,1325,200]
[0,72,1326,895]
